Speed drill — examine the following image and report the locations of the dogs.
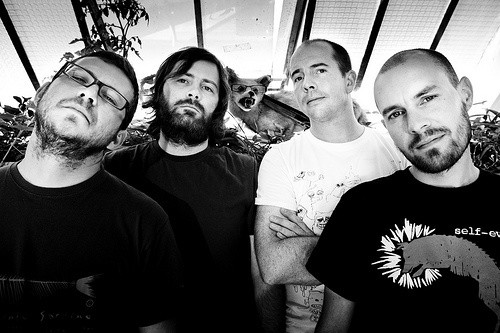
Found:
[226,66,302,142]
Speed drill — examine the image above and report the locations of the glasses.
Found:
[231,84,267,94]
[52,61,130,111]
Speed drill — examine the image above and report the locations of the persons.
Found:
[102,47,285,333]
[305,47,500,333]
[252,39,413,333]
[0,50,172,333]
